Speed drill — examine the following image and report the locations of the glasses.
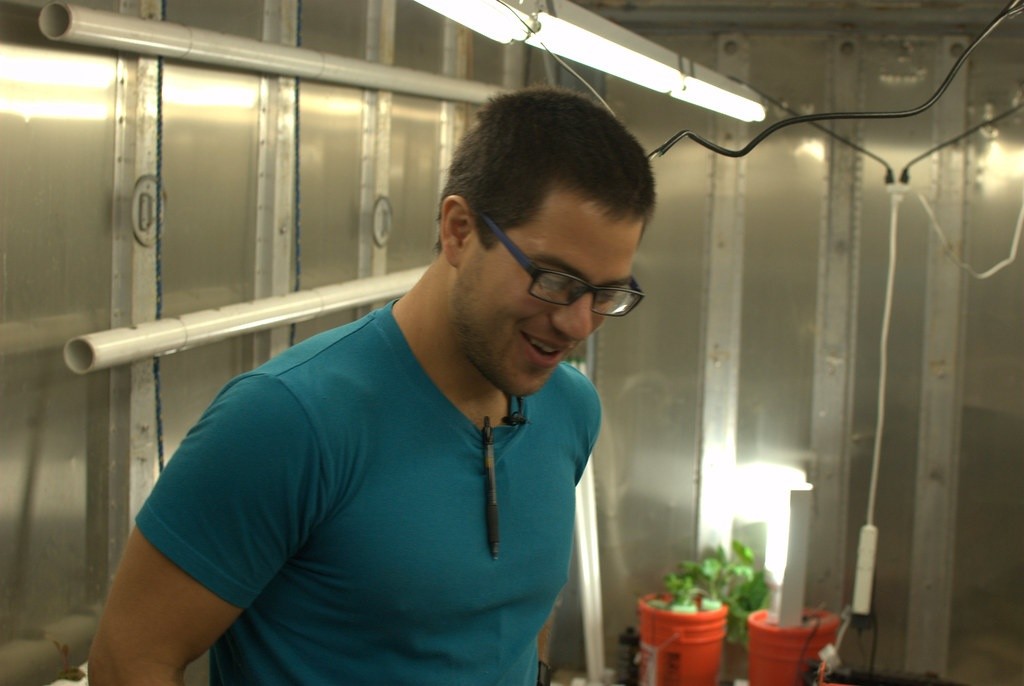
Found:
[477,211,645,318]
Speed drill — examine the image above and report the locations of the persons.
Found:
[86,80,660,686]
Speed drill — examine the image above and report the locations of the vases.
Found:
[745,607,841,685]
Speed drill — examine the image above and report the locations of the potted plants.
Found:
[638,542,770,686]
